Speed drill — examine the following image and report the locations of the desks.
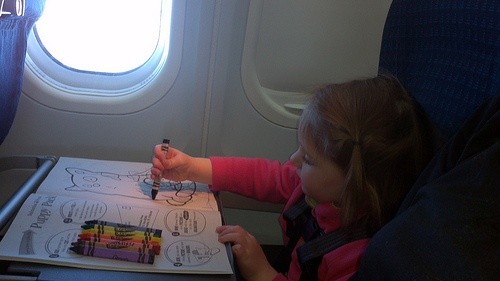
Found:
[0,155,237,281]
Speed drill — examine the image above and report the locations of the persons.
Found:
[150,73,425,281]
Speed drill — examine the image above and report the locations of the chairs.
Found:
[355,0,500,280]
